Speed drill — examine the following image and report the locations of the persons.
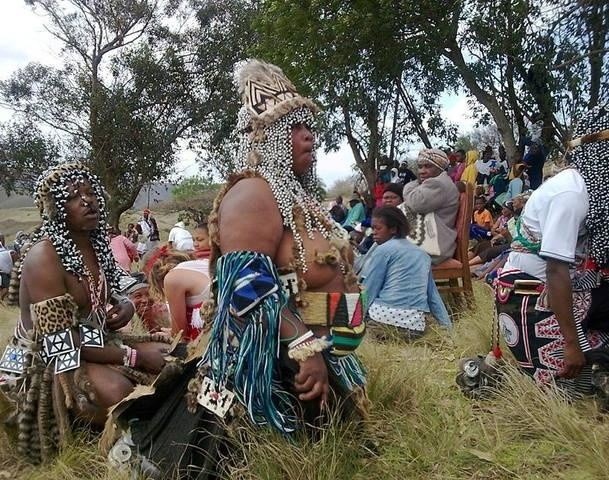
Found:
[2,213,217,395]
[1,158,188,467]
[202,54,377,462]
[495,96,608,400]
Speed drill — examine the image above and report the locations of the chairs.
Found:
[426,181,478,323]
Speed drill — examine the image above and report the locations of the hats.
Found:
[144,208,151,214]
[230,56,318,132]
[124,271,151,295]
[381,183,403,201]
[501,200,514,212]
[417,148,451,173]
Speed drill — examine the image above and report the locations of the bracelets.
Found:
[122,345,140,371]
[281,339,329,361]
[287,329,315,349]
[123,296,139,312]
